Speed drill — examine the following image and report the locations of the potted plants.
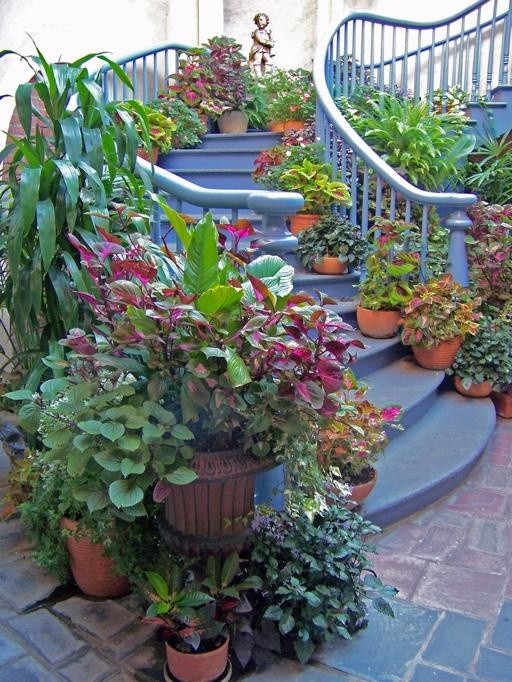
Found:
[1,37,511,680]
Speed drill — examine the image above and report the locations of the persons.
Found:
[249,13,275,80]
